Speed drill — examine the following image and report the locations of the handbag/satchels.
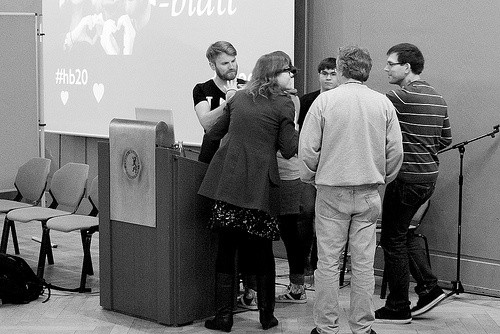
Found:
[0,254,43,306]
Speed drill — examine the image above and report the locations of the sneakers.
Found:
[236,289,258,310]
[276,283,307,303]
[412,284,446,315]
[374,304,413,323]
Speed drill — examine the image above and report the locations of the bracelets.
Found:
[226,88,237,93]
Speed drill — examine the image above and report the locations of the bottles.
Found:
[178,140,185,157]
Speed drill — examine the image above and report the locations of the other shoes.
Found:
[310,327,319,334]
[370,329,376,334]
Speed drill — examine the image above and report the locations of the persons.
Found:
[297,56,341,134]
[62,0,156,57]
[297,47,403,334]
[370,43,452,323]
[198,50,298,333]
[236,87,307,310]
[193,40,249,134]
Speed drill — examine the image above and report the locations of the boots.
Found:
[205,286,234,332]
[258,284,279,329]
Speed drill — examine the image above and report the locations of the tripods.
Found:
[436,124,500,299]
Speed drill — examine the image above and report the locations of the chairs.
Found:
[0,157,100,293]
[338,199,432,299]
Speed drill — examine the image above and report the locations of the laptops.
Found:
[135,107,200,155]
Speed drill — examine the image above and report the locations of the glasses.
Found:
[385,61,407,68]
[275,65,291,75]
